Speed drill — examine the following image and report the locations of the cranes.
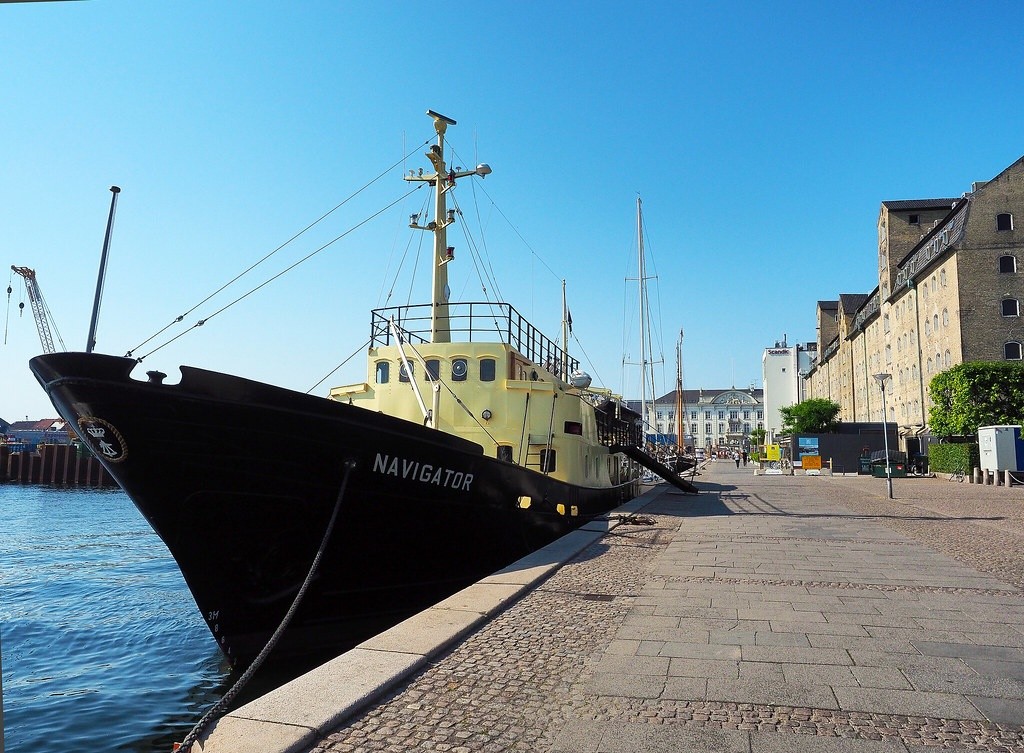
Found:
[8,263,57,354]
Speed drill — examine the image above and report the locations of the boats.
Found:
[26,111,697,696]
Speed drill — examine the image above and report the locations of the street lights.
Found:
[871,373,898,496]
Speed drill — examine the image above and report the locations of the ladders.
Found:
[525,433,554,475]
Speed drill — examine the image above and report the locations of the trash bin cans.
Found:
[871,449,907,478]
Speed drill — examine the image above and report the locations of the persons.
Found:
[710,447,716,462]
[741,450,747,468]
[734,449,740,470]
